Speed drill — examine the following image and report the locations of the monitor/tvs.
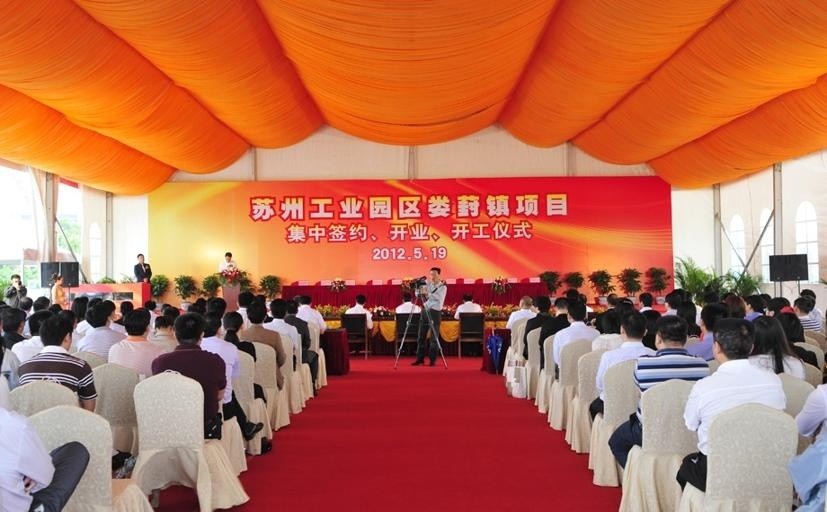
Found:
[769,255,809,282]
[41,262,79,287]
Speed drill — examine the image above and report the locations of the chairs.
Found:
[342,315,367,360]
[396,313,422,362]
[459,312,485,359]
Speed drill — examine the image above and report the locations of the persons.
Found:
[394,292,421,322]
[217,252,237,276]
[133,253,152,284]
[453,292,482,356]
[344,293,373,355]
[410,268,447,366]
[507,290,827,512]
[0,274,326,511]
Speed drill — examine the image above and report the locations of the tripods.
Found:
[394,291,448,370]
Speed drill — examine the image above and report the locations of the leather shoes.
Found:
[410,362,424,365]
[261,442,272,455]
[244,423,263,441]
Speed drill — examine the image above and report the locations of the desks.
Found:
[281,281,550,315]
[321,320,511,357]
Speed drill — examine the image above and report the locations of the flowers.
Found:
[217,269,243,287]
[403,277,414,293]
[491,276,509,296]
[373,305,393,317]
[330,278,348,293]
[441,304,456,317]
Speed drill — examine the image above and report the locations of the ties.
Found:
[141,264,146,273]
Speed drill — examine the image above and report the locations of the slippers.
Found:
[112,449,131,471]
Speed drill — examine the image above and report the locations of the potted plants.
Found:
[258,275,282,316]
[646,266,669,304]
[174,275,195,311]
[150,276,169,309]
[589,270,616,307]
[542,269,561,304]
[618,268,643,304]
